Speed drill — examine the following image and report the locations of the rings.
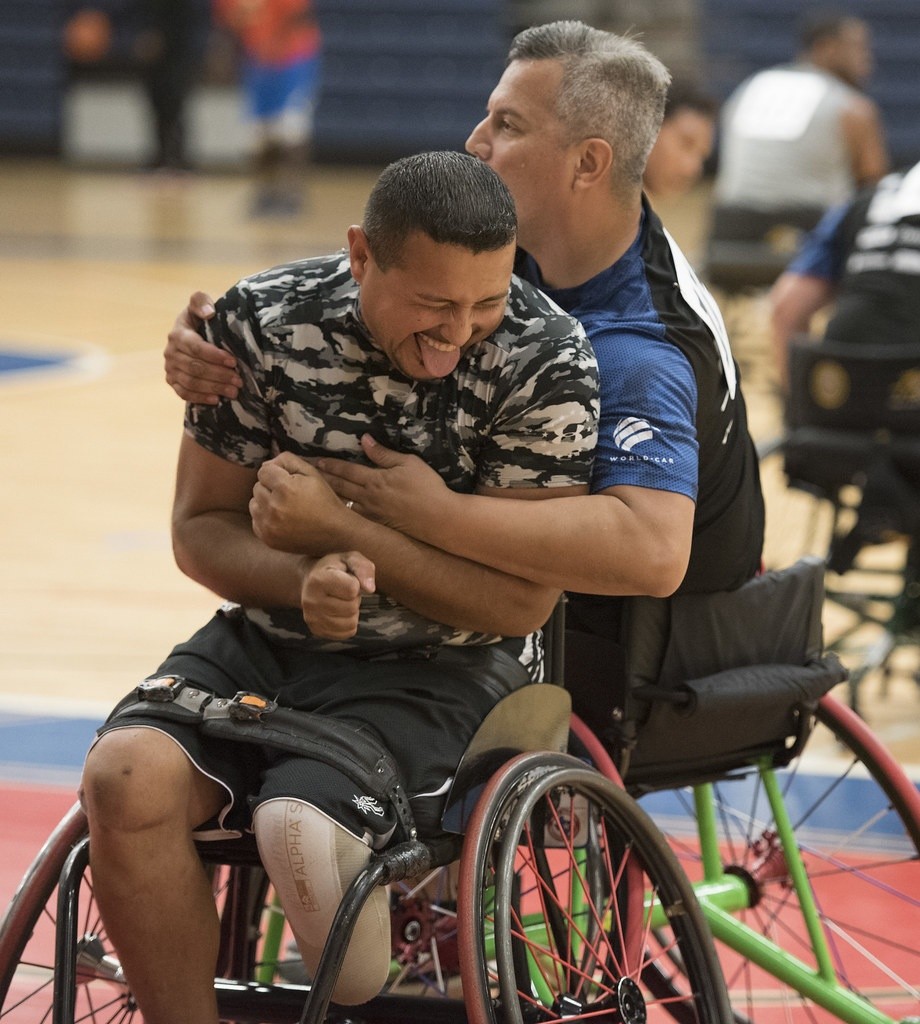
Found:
[347,500,353,509]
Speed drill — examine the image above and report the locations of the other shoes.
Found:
[276,918,463,989]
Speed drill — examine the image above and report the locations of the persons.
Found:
[767,163,920,645]
[214,0,323,216]
[78,152,602,1024]
[704,13,886,295]
[641,76,715,196]
[165,21,765,645]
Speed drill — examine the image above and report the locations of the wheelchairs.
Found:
[752,339,920,716]
[0,595,737,1023]
[224,555,919,1024]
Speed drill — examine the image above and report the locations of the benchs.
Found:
[700,0,920,171]
[1,2,504,163]
